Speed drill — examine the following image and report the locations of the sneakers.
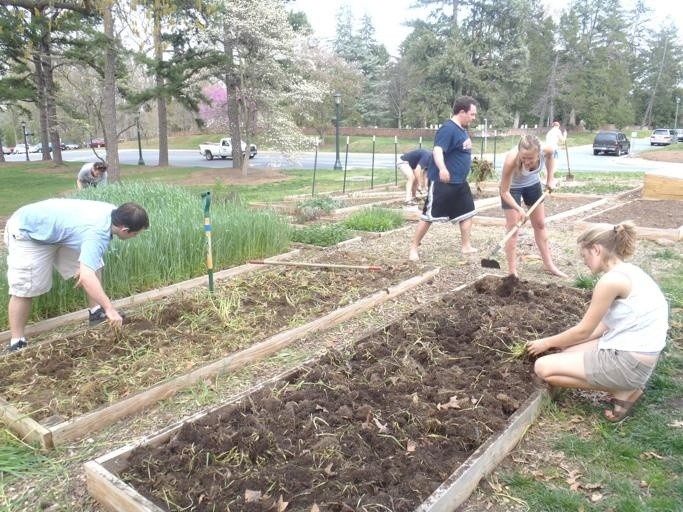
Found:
[9,339,27,351]
[87,308,109,328]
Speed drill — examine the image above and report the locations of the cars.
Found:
[673,128,683,141]
[1,137,80,155]
[84,138,105,147]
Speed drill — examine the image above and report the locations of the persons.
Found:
[77,162,108,190]
[409,96,479,261]
[526,219,668,423]
[544,122,567,166]
[397,149,432,205]
[3,197,149,350]
[499,133,570,279]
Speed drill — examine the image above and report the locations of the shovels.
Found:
[565,141,574,182]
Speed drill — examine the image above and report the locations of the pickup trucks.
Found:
[197,138,256,161]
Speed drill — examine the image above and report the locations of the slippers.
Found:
[603,385,647,422]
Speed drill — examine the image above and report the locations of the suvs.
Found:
[591,130,630,157]
[649,127,678,145]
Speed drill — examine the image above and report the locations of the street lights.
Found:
[332,90,345,169]
[673,97,680,128]
[20,119,30,160]
